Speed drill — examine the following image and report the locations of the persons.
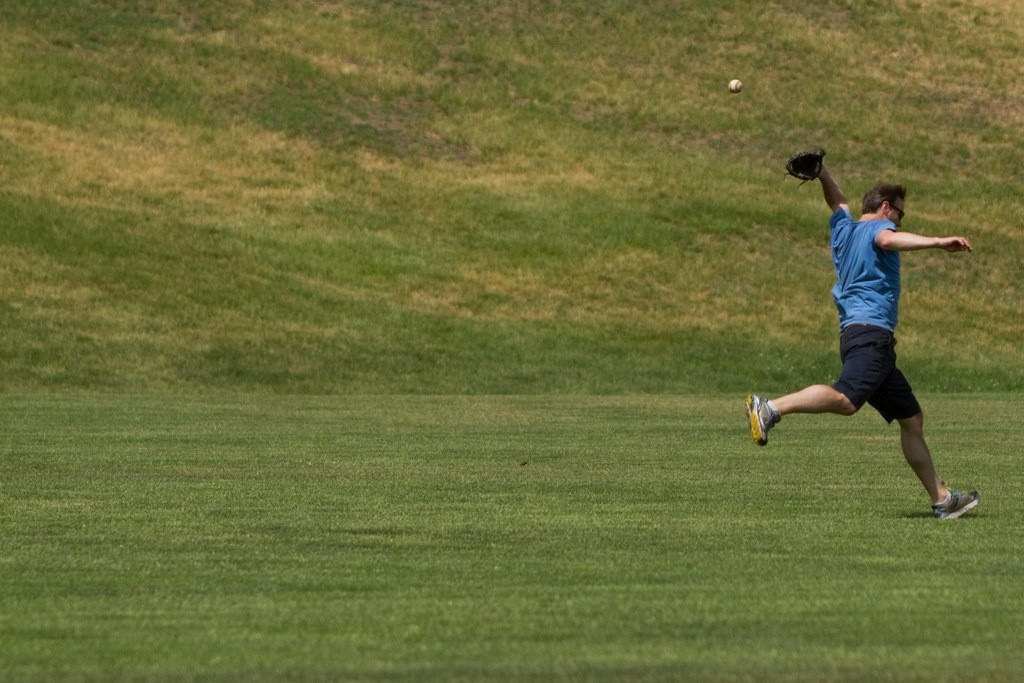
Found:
[746,149,981,520]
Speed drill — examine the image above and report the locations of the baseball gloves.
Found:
[784,148,826,188]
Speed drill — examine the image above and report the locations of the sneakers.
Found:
[932,488,980,520]
[744,394,782,445]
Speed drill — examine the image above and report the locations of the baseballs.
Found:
[728,79,743,93]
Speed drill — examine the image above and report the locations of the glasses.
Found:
[880,199,905,220]
